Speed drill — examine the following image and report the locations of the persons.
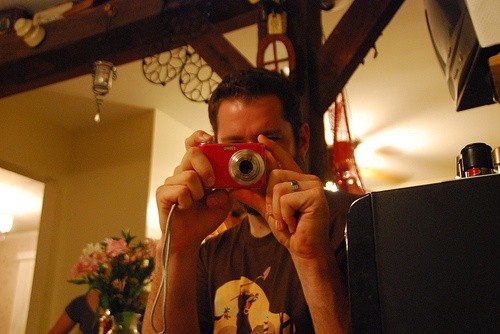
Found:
[141,68,370,334]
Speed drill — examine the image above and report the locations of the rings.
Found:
[289,180,299,191]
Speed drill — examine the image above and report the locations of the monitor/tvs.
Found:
[424,0,479,105]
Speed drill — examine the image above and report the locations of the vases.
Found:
[102,313,142,334]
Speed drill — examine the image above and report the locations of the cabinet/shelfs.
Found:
[489,52,500,105]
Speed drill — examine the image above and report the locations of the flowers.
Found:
[65,226,157,329]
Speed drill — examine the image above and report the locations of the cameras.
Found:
[198,143,267,190]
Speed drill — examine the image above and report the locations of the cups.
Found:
[461,142,494,176]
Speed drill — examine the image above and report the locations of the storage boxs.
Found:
[346,172,499,333]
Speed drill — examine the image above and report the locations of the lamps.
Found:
[88,60,119,123]
